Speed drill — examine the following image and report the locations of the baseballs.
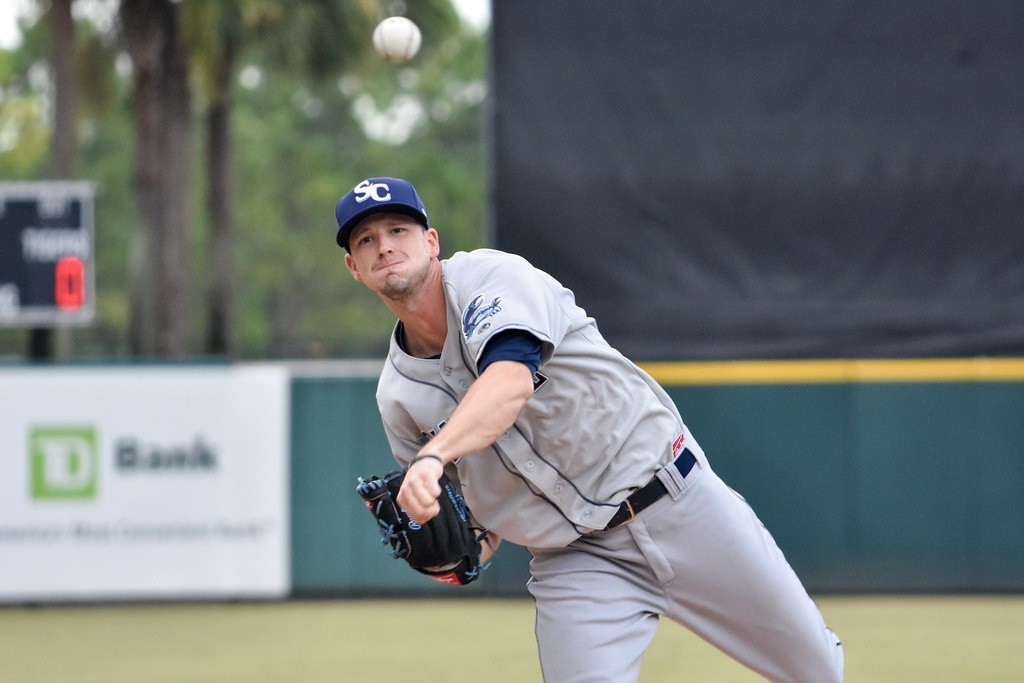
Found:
[371,15,423,64]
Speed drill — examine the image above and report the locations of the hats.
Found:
[335,177,429,254]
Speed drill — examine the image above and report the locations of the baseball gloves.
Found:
[355,463,483,586]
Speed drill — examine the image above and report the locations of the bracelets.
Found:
[408,455,443,468]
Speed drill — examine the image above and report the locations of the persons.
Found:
[334,177,845,683]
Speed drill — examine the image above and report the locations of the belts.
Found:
[594,448,697,532]
